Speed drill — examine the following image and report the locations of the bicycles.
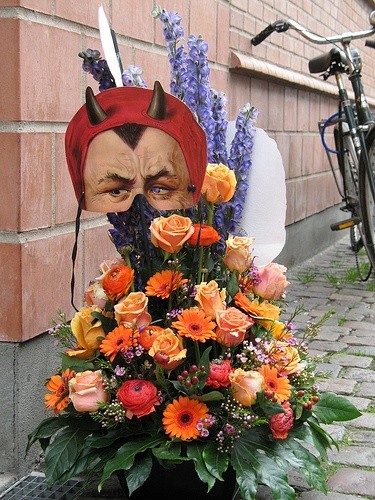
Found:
[250,10,375,283]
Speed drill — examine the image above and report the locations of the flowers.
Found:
[25,5,362,498]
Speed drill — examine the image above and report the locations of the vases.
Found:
[119,450,249,500]
